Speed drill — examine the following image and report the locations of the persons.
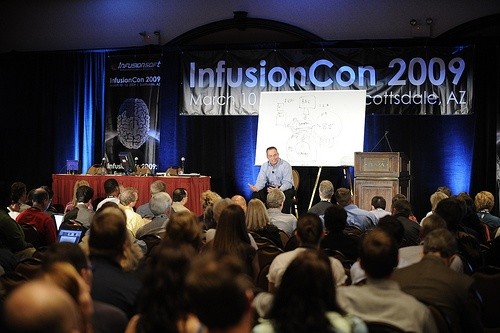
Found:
[0,178,500,332]
[248,147,294,214]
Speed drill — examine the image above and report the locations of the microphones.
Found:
[181,157,185,167]
[101,157,106,166]
[135,157,138,166]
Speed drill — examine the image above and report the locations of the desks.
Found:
[52,174,210,215]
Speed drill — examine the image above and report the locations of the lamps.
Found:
[410,17,432,38]
[139,31,160,44]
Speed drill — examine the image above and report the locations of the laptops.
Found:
[55,225,84,245]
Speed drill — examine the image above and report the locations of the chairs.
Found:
[292,170,300,219]
[88,164,181,175]
